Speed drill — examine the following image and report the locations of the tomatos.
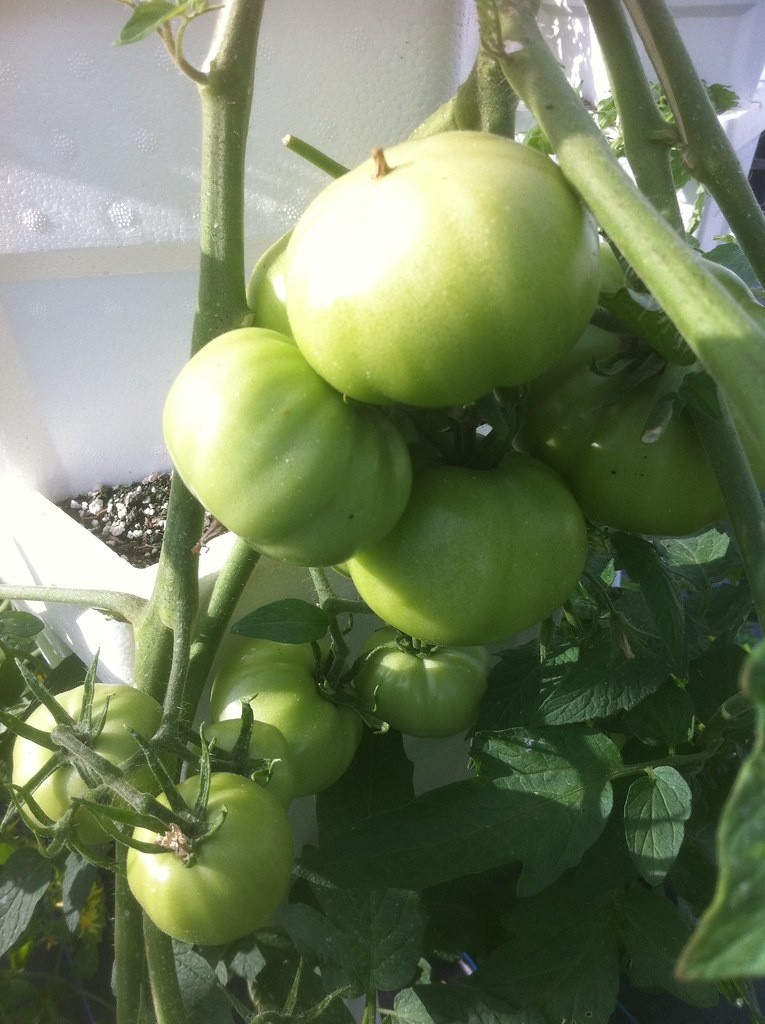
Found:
[11,126,765,949]
